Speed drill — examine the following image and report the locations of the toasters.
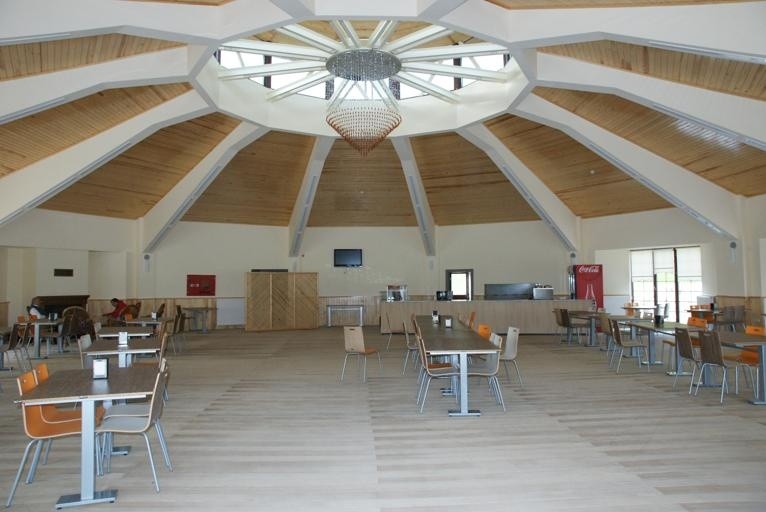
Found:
[436,291,452,301]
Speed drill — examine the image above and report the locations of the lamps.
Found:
[320,47,403,158]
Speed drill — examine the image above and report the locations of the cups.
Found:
[655,315,664,324]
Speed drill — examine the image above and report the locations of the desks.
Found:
[326,304,364,326]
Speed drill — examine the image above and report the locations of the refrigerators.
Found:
[568,264,605,333]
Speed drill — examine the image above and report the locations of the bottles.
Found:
[584,282,597,310]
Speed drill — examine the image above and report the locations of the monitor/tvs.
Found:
[334,249,362,266]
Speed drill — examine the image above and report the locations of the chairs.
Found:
[0,297,220,511]
[338,325,383,386]
[551,301,766,409]
[384,311,526,418]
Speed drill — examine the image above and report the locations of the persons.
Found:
[106,298,127,322]
[28,296,49,320]
